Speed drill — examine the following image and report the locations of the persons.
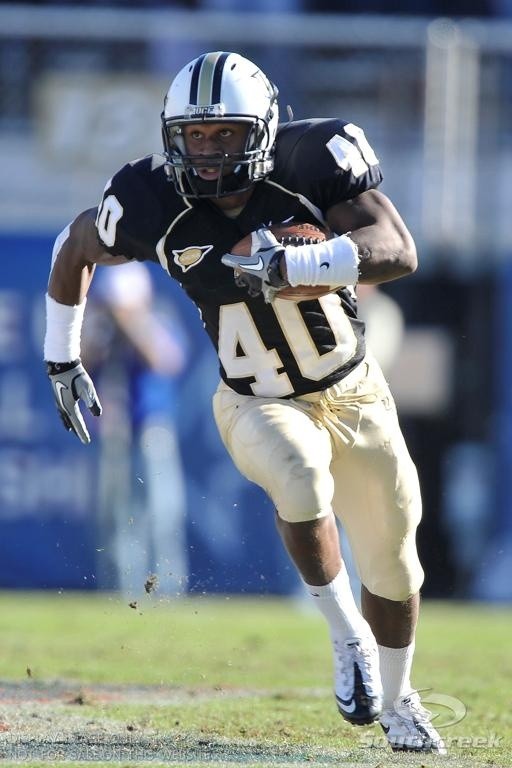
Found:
[42,51,448,758]
[78,258,191,599]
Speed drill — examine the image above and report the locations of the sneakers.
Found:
[375,686,445,756]
[333,619,384,724]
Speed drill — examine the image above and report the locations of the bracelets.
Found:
[42,294,88,364]
[282,230,363,290]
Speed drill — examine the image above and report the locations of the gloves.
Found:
[220,229,290,305]
[47,359,102,444]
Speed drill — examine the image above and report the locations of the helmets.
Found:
[161,51,280,202]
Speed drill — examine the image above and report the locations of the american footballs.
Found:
[231,222,345,301]
